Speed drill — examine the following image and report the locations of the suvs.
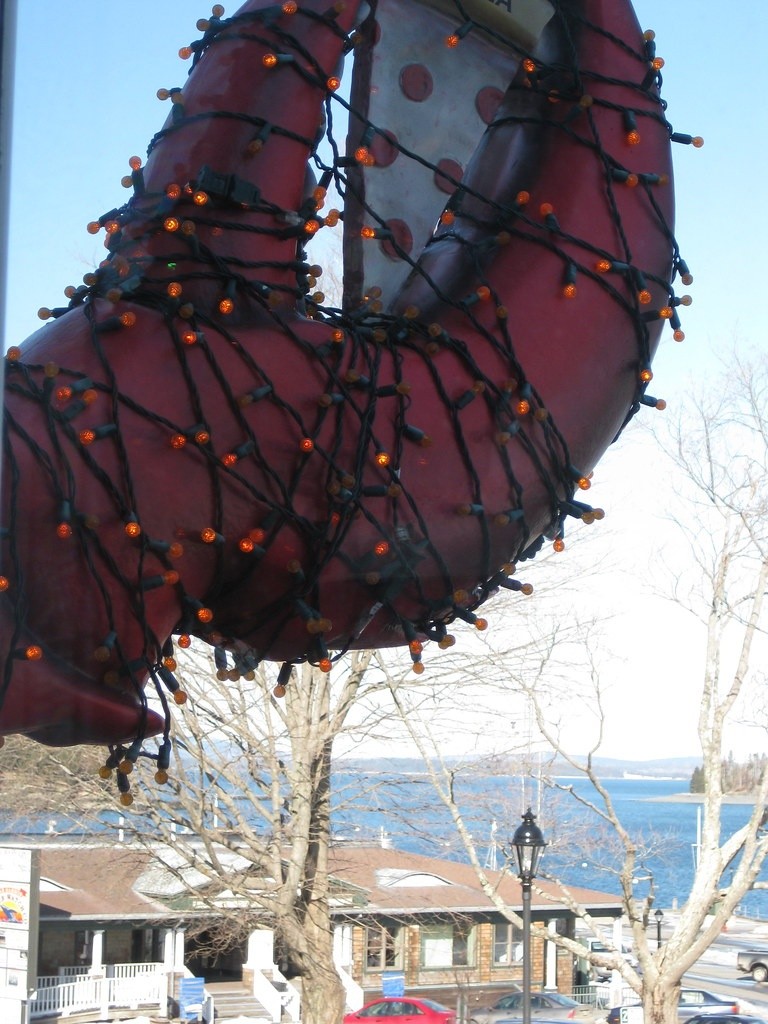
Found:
[574,936,640,982]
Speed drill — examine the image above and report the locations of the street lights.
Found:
[653,908,664,948]
[509,806,550,1024]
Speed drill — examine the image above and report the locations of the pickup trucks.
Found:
[736,948,768,983]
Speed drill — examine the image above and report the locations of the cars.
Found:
[606,989,738,1024]
[343,996,458,1024]
[468,991,594,1024]
[683,1012,766,1024]
